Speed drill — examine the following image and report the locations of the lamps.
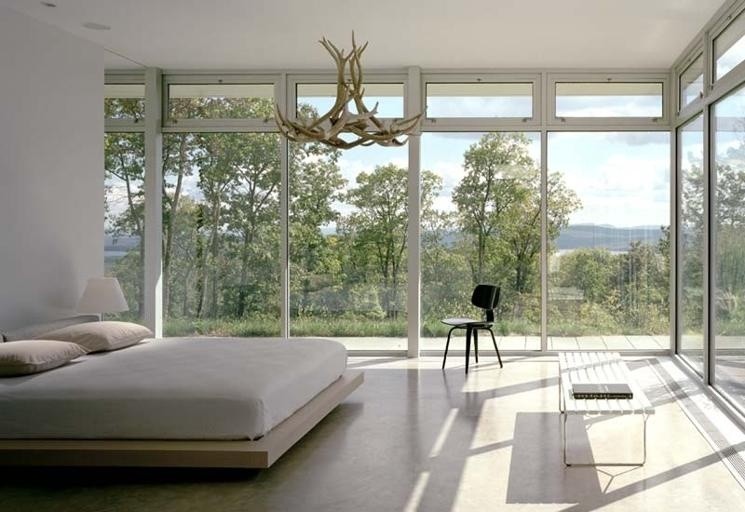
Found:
[76,277,130,323]
[273,28,428,150]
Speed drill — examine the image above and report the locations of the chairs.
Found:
[441,284,503,374]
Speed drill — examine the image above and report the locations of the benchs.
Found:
[558,352,655,467]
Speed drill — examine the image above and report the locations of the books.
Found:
[572,383,634,399]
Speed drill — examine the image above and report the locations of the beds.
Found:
[0,314,364,469]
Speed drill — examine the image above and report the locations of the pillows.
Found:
[34,321,156,353]
[1,340,92,374]
